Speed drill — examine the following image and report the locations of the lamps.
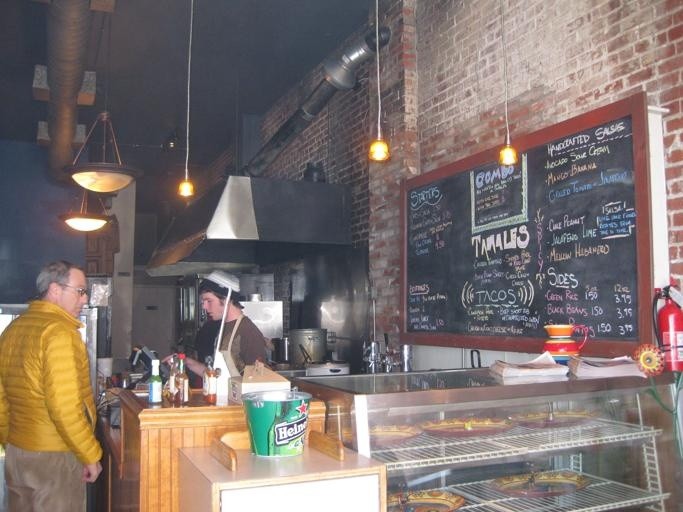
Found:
[68,10,143,194]
[367,0,391,162]
[498,1,517,166]
[176,0,195,199]
[57,133,113,232]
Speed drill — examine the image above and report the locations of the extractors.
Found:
[143,174,355,279]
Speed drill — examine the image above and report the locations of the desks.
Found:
[177,437,388,512]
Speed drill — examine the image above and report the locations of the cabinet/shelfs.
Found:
[286,367,683,511]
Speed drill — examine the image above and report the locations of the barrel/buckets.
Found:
[240,386,313,458]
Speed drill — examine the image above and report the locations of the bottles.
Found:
[211,351,231,405]
[149,358,163,404]
[164,351,189,403]
[201,354,217,404]
[104,369,129,389]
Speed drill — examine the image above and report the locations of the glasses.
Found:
[60,283,87,296]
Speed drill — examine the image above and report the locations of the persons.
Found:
[161,268,268,405]
[0,258,105,512]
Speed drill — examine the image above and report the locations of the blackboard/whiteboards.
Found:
[399,92,654,357]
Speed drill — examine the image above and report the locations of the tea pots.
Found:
[542,322,588,364]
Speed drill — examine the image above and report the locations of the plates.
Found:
[486,471,593,498]
[367,424,424,446]
[383,490,465,511]
[510,408,597,430]
[420,417,514,437]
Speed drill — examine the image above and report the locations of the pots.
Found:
[269,337,288,363]
[287,327,326,364]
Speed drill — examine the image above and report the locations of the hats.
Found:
[206,271,240,293]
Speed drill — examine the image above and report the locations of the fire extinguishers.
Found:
[653,275,683,371]
